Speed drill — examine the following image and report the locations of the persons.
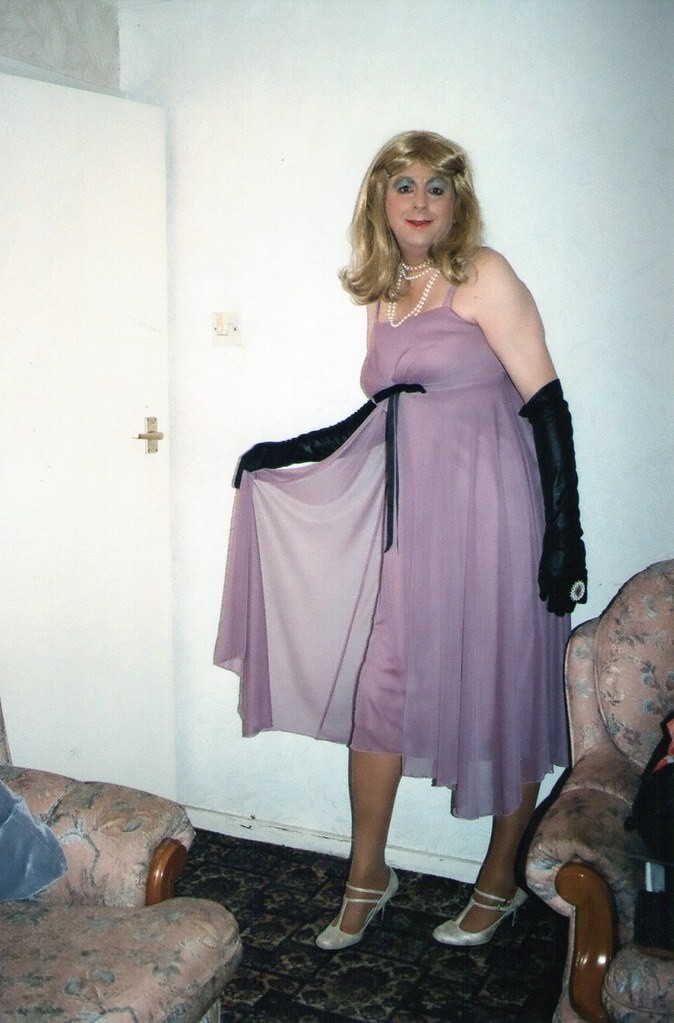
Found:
[213,130,588,950]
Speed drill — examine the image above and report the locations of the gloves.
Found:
[233,399,377,489]
[517,378,588,617]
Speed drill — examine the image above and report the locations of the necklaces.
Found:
[388,261,439,327]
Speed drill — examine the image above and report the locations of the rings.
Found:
[570,581,585,601]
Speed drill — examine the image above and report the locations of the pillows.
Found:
[0,779,67,903]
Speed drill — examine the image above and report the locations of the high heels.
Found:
[315,865,399,951]
[431,885,529,946]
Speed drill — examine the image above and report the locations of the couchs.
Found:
[512,557,674,1023]
[0,767,242,1023]
